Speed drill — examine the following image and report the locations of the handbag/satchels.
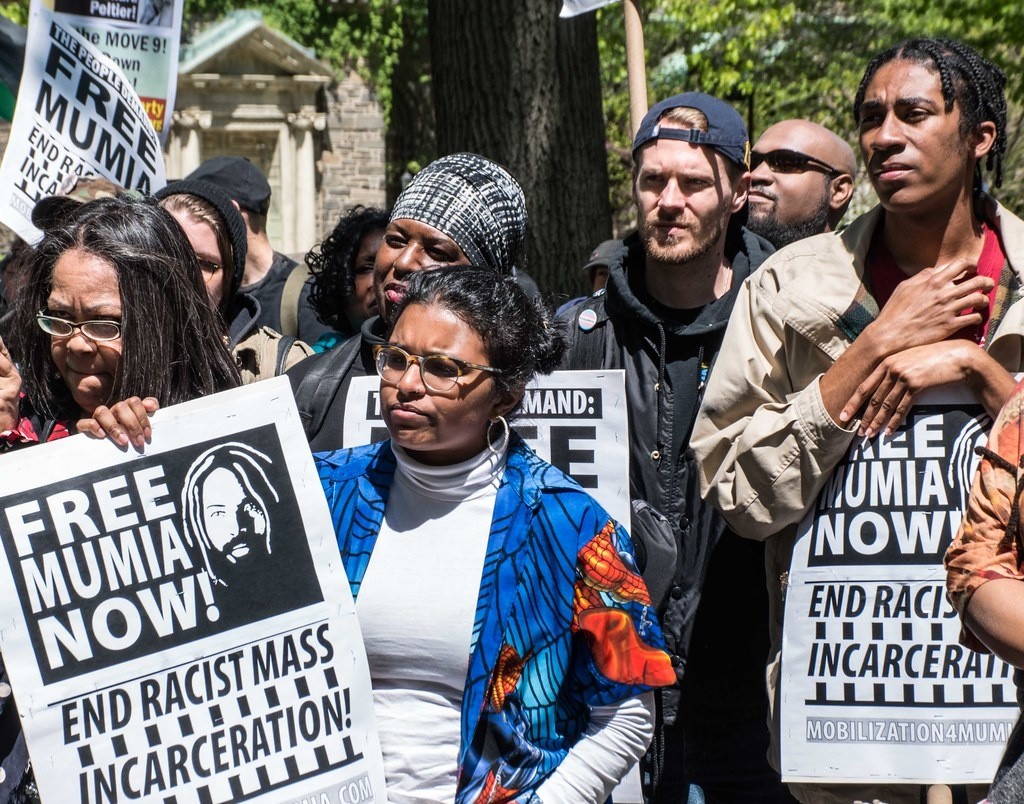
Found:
[630,479,677,616]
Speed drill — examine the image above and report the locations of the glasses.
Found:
[35,306,123,341]
[372,342,502,393]
[196,257,226,284]
[746,149,840,181]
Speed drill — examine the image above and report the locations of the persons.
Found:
[304,204,393,353]
[75,265,677,804]
[550,93,781,804]
[691,39,1024,804]
[942,375,1024,669]
[0,179,130,314]
[284,154,529,451]
[150,181,316,385]
[186,157,334,345]
[748,119,858,247]
[0,198,241,452]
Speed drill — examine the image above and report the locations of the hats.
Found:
[29,176,126,228]
[631,92,748,172]
[582,240,625,272]
[152,179,247,302]
[167,155,271,214]
